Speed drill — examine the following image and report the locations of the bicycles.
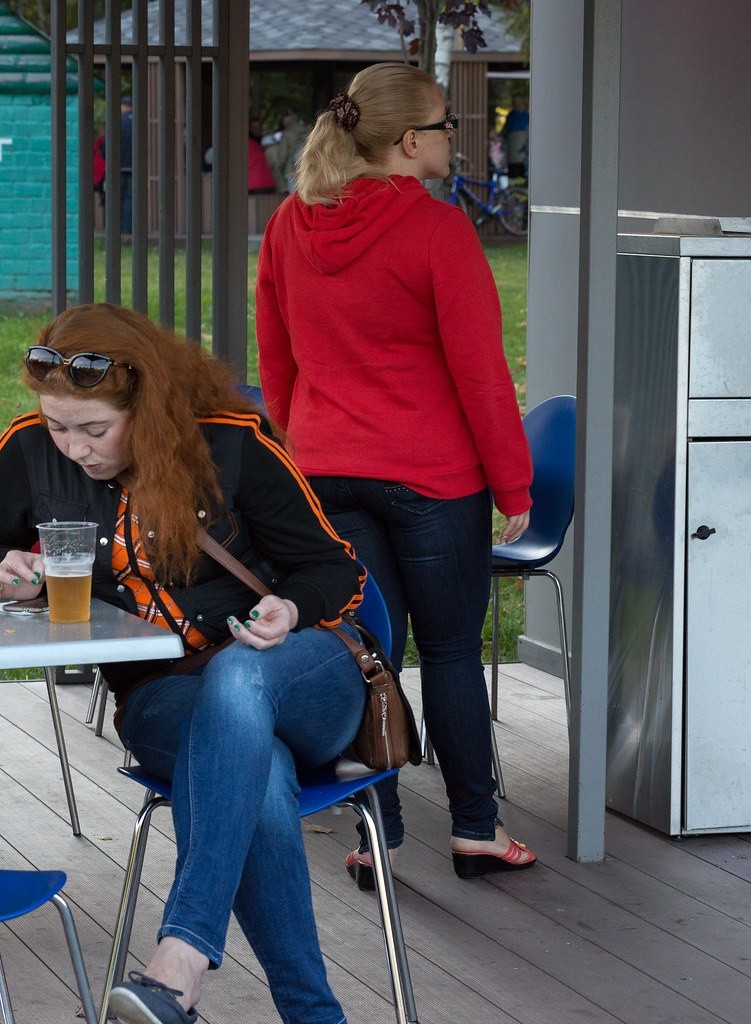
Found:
[427,152,528,237]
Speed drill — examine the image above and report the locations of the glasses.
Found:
[394,114,458,146]
[26,345,137,387]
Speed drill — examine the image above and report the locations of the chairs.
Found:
[422,394,579,800]
[0,870,98,1024]
[99,554,420,1024]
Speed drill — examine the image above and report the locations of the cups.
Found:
[37,522,99,624]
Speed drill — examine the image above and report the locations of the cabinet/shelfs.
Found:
[606,232,751,837]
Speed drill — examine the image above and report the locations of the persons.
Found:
[0,304,366,1024]
[94,96,307,235]
[489,93,528,180]
[257,62,538,893]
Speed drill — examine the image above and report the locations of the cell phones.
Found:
[3,597,50,613]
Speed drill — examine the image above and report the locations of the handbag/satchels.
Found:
[355,660,422,771]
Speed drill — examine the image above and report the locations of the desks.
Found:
[0,596,185,669]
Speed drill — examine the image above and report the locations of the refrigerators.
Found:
[605,234,751,838]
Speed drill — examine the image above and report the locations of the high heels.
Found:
[343,851,375,892]
[452,836,537,877]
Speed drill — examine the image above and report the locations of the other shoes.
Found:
[110,971,196,1024]
